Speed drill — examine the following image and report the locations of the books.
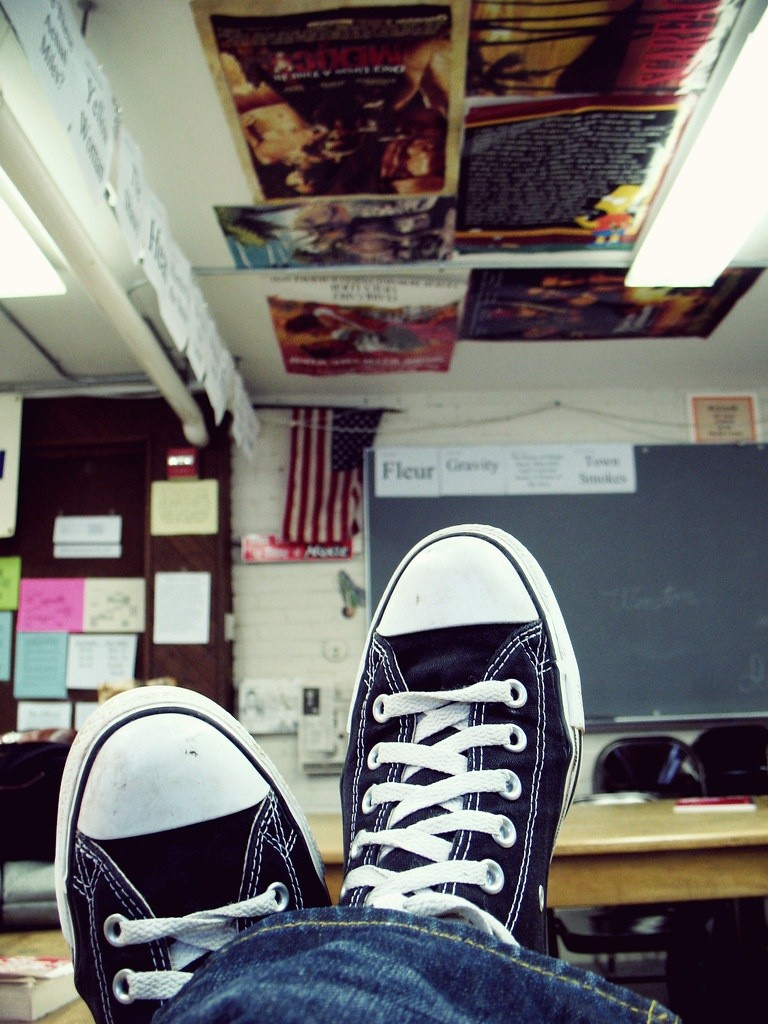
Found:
[1,956,81,1020]
[672,794,756,812]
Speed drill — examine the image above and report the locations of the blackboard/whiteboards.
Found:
[362,440,768,727]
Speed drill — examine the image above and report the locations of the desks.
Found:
[0,928,95,1024]
[301,794,768,912]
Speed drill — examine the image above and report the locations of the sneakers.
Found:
[55,685,334,1024]
[339,524,585,956]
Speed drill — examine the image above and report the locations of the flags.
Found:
[281,402,388,547]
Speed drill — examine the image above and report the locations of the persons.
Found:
[56,521,689,1024]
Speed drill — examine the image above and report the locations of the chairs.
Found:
[554,724,768,1024]
